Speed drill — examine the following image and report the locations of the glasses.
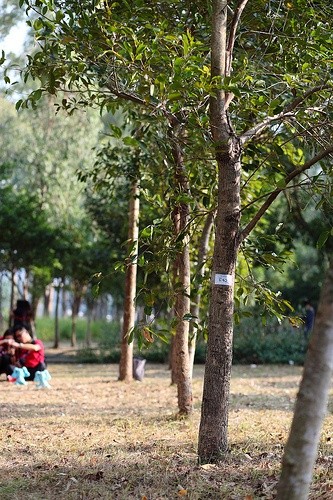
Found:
[14,335,21,339]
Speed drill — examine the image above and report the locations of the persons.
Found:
[8,323,46,381]
[13,299,32,332]
[0,329,15,381]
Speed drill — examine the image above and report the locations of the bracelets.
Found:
[19,342,23,349]
